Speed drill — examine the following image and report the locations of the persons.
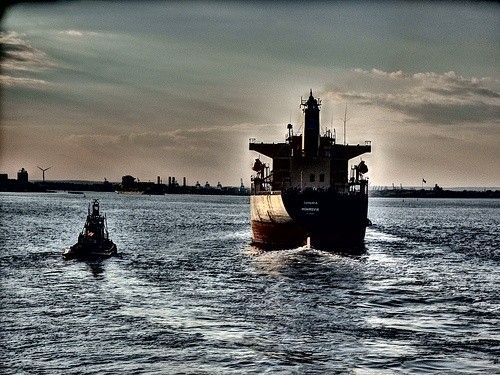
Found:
[290,185,329,193]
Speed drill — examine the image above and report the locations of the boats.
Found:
[246,86,371,256]
[63,195,120,266]
